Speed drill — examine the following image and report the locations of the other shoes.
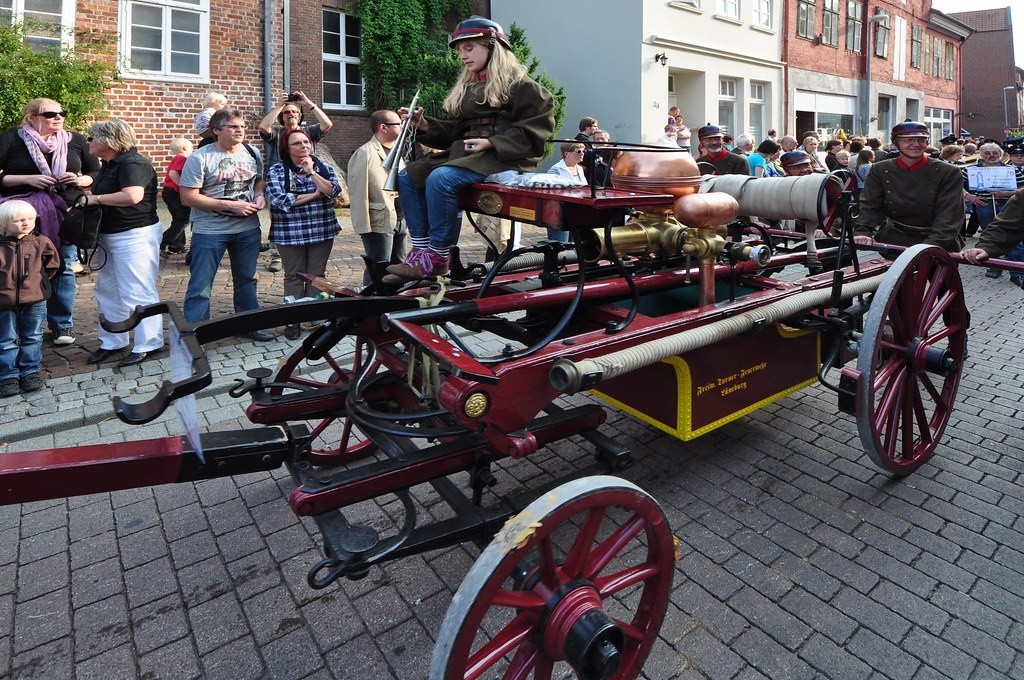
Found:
[160,247,187,259]
[381,247,451,284]
[260,244,270,252]
[985,269,1001,278]
[948,332,967,359]
[269,255,282,272]
[1010,276,1022,286]
[199,323,300,357]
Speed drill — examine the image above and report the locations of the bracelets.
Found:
[97,195,102,205]
[306,171,315,178]
[309,104,317,111]
[254,194,264,198]
[79,176,84,186]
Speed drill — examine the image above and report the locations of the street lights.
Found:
[1003,85,1015,139]
[862,14,891,138]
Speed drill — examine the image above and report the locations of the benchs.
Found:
[454,139,687,225]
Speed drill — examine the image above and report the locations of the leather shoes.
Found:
[88,348,158,366]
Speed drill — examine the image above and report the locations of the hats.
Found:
[939,135,958,144]
[1003,137,1024,154]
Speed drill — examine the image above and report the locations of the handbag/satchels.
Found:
[58,193,107,271]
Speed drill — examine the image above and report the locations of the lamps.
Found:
[655,52,668,67]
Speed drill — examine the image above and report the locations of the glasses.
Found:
[287,140,312,147]
[384,122,402,127]
[223,125,248,129]
[569,150,585,154]
[281,109,301,114]
[88,137,93,142]
[32,112,67,118]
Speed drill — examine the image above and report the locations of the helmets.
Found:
[780,151,811,167]
[449,17,512,51]
[698,126,723,138]
[891,122,929,137]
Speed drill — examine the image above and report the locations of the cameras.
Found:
[285,94,299,102]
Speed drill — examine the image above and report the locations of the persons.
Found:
[348,110,408,287]
[266,128,342,340]
[67,120,164,367]
[160,93,274,359]
[259,91,332,272]
[658,107,691,151]
[0,98,100,344]
[853,117,970,361]
[382,16,554,286]
[696,116,1024,289]
[474,117,622,264]
[0,200,59,397]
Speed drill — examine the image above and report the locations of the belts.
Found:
[887,217,932,237]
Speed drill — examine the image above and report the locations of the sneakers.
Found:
[0,327,75,397]
